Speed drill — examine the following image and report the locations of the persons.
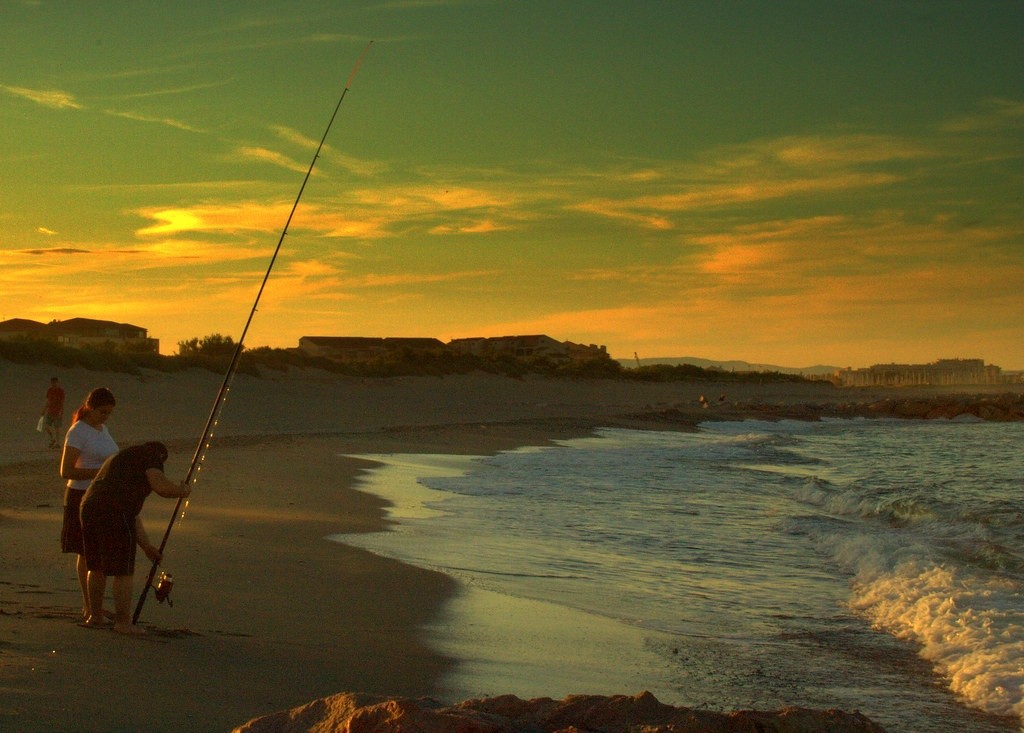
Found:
[41,378,65,449]
[79,441,192,633]
[59,387,121,623]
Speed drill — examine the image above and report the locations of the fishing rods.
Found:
[128,32,386,632]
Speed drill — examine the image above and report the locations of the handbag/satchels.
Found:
[37,415,44,433]
